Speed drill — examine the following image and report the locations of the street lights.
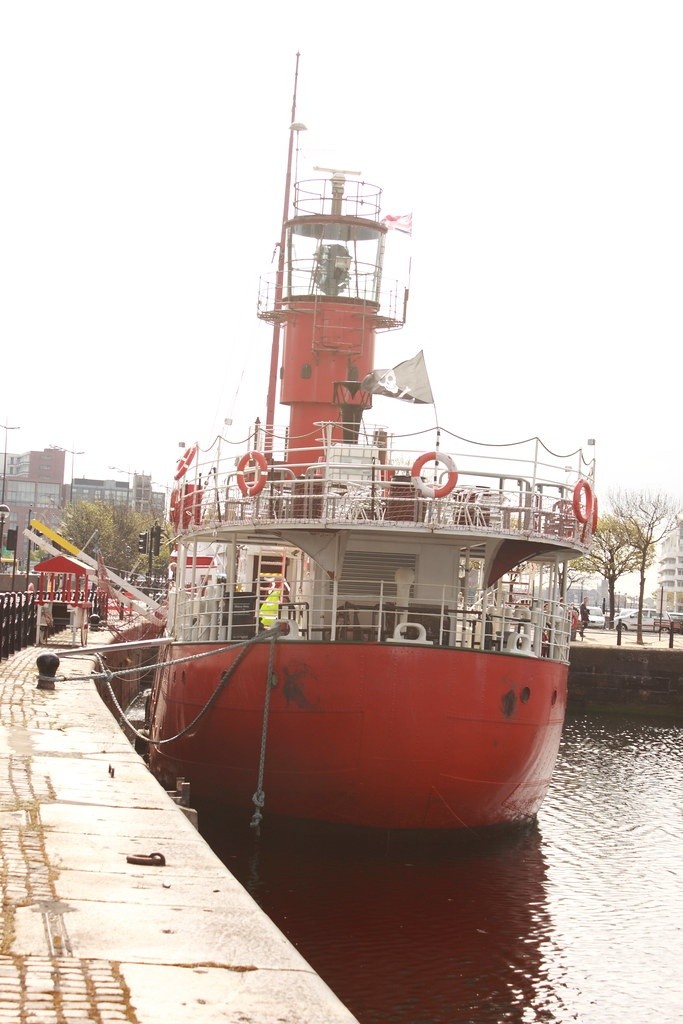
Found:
[0,416,23,505]
[0,504,11,573]
[65,447,86,509]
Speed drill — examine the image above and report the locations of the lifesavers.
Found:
[169,489,177,521]
[542,632,547,656]
[573,480,594,523]
[236,451,267,496]
[591,496,599,535]
[411,452,457,499]
[174,445,196,479]
[569,612,576,627]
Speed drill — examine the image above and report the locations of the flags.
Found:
[362,349,435,405]
[224,418,233,425]
[588,439,596,445]
[382,211,411,235]
[178,442,186,447]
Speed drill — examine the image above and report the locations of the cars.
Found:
[612,608,672,635]
[619,609,633,618]
[666,611,683,635]
[577,606,606,630]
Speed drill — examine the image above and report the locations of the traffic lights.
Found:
[152,526,166,556]
[137,532,148,555]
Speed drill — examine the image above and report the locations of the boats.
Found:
[141,50,605,836]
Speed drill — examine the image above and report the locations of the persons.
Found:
[260,578,296,629]
[580,596,591,637]
[455,589,578,634]
